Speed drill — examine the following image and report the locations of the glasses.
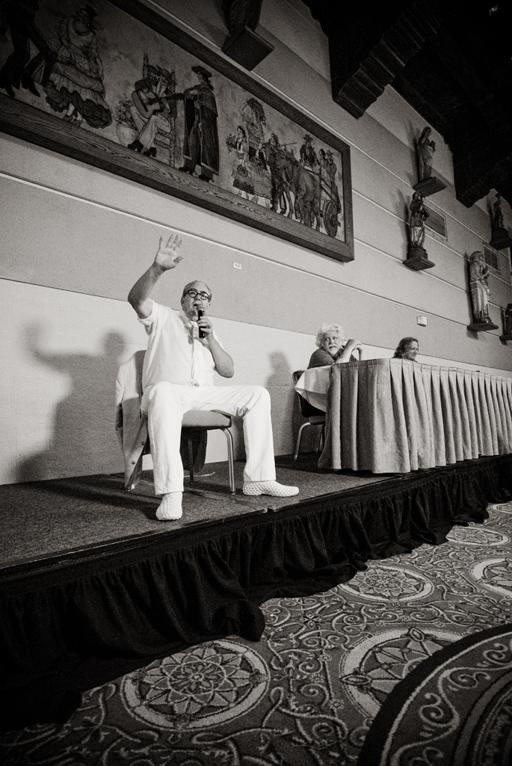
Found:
[183,290,211,300]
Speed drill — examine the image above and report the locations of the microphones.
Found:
[197,304,205,338]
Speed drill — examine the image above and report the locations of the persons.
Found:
[408,189,429,247]
[468,250,493,322]
[392,337,419,361]
[491,192,506,228]
[306,323,367,368]
[418,125,433,177]
[126,232,300,523]
[504,303,511,331]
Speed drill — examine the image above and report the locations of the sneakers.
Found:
[156,492,183,521]
[242,480,299,497]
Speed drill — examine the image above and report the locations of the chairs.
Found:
[112,350,235,496]
[294,370,324,460]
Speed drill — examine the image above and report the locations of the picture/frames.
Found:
[0,0,354,262]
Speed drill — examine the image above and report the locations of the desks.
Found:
[294,359,511,474]
